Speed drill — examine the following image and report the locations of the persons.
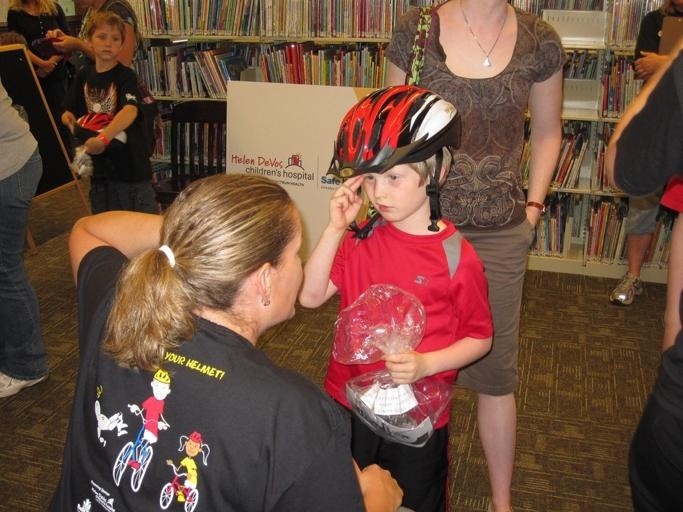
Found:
[300,88,493,512]
[629,167,682,509]
[610,0,683,306]
[386,1,569,512]
[1,0,135,397]
[49,173,403,512]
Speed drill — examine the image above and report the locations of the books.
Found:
[134,2,683,265]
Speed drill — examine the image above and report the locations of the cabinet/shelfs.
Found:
[138,1,677,286]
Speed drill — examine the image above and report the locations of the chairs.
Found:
[156,100,226,211]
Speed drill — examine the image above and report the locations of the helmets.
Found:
[325,83,464,179]
[71,111,128,152]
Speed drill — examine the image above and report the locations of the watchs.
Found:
[526,201,546,215]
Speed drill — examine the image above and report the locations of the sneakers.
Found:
[0,370,50,400]
[607,270,646,308]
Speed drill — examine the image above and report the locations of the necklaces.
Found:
[459,1,508,67]
